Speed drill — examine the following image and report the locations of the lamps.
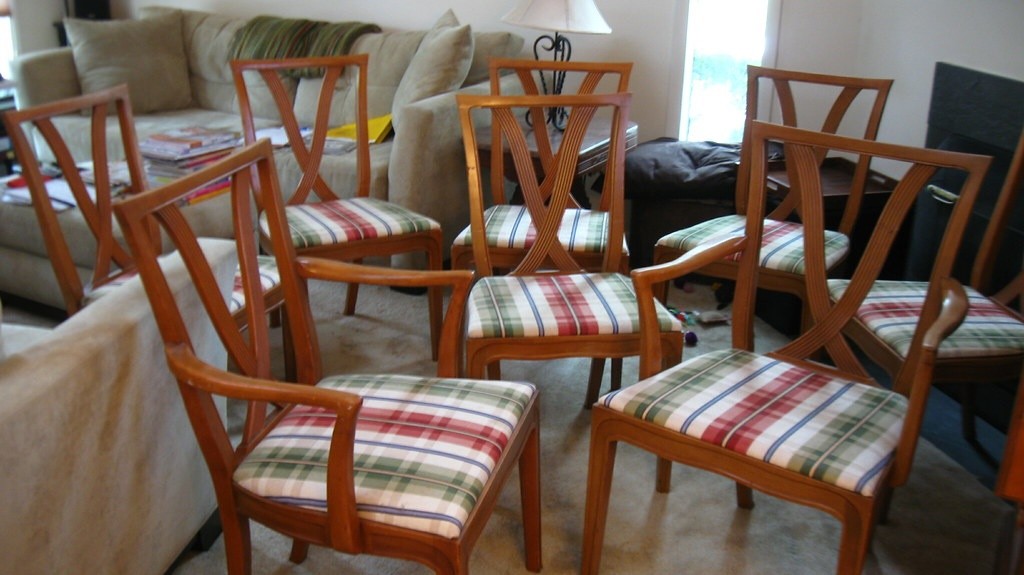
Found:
[500,0,612,132]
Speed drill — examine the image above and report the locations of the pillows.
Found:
[390,8,474,134]
[63,7,196,116]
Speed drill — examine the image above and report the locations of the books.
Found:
[236,114,391,153]
[139,127,239,204]
[13,146,152,187]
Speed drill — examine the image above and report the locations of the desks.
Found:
[459,113,639,210]
[754,157,915,359]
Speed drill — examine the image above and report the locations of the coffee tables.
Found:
[0,162,262,309]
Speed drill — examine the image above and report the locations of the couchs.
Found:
[11,8,525,288]
[0,237,237,575]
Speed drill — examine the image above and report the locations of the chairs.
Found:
[0,55,1024,575]
